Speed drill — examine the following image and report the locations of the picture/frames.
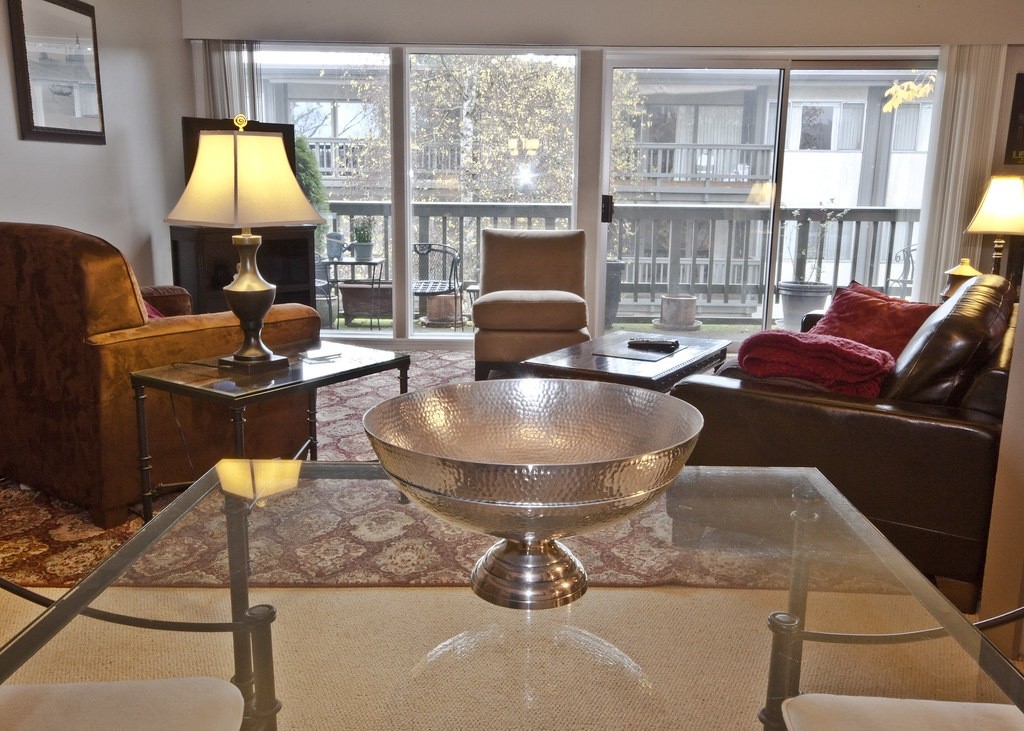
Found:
[8,0,108,149]
[1003,72,1024,165]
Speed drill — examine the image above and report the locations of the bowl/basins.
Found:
[361,378,704,610]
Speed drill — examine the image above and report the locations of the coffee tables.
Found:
[128,340,413,532]
[518,327,737,391]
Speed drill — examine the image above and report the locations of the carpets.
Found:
[0,345,939,595]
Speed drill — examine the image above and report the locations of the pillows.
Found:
[848,280,928,303]
[809,287,943,363]
[143,298,166,319]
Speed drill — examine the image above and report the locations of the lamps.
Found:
[216,458,304,508]
[962,173,1024,275]
[161,112,326,375]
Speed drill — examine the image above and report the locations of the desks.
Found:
[321,257,386,332]
[0,454,1024,731]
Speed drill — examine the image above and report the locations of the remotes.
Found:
[628,337,679,352]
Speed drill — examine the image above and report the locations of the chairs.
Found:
[758,606,1024,731]
[315,252,332,329]
[696,153,716,180]
[883,243,917,300]
[411,242,465,333]
[471,229,591,383]
[0,578,284,731]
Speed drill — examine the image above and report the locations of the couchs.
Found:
[0,221,318,528]
[667,274,1021,589]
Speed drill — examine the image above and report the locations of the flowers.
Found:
[777,197,852,284]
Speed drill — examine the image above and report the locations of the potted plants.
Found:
[351,215,377,261]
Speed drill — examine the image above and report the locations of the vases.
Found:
[650,294,704,331]
[419,292,469,328]
[315,294,337,326]
[779,281,833,330]
[335,281,393,326]
[605,258,626,330]
